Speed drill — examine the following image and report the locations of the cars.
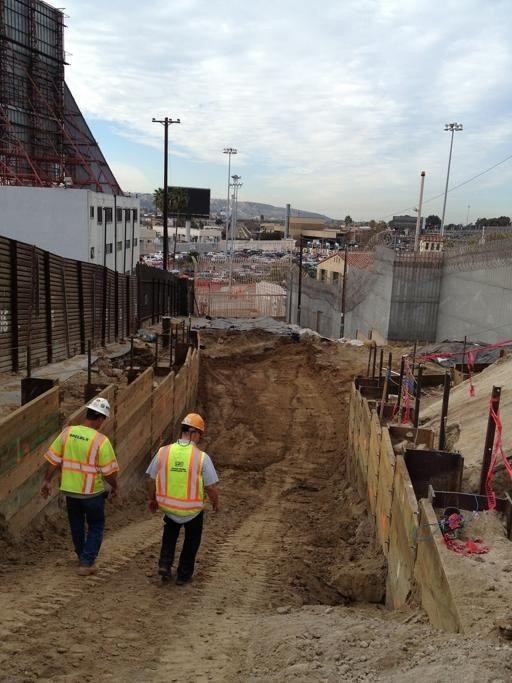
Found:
[140,249,327,285]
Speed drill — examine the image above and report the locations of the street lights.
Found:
[439,123,464,239]
[222,147,244,298]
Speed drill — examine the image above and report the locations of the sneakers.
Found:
[157,563,173,582]
[77,564,99,577]
[175,576,194,586]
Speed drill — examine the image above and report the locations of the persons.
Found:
[145,412,224,586]
[41,396,121,576]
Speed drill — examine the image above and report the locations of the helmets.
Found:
[84,398,111,418]
[181,413,205,433]
